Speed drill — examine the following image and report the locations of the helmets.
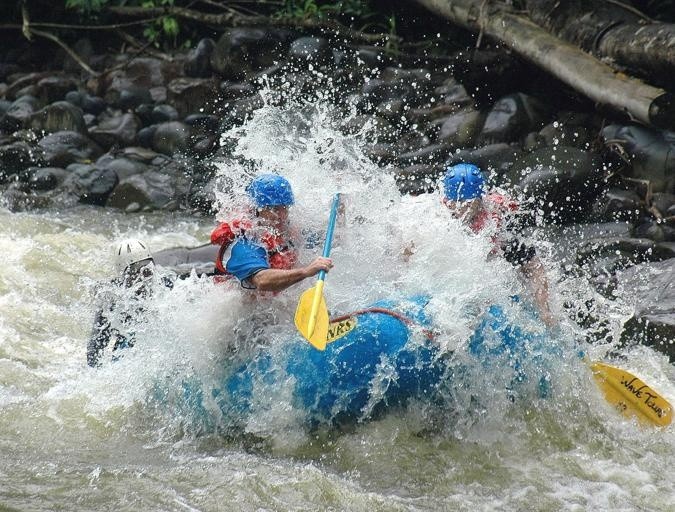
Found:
[445,164,484,201]
[245,175,294,208]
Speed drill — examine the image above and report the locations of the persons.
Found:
[214,175,347,297]
[440,161,556,327]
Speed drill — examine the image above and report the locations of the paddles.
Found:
[578,351,674,433]
[294,193,340,351]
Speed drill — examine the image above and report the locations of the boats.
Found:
[84,224,587,444]
